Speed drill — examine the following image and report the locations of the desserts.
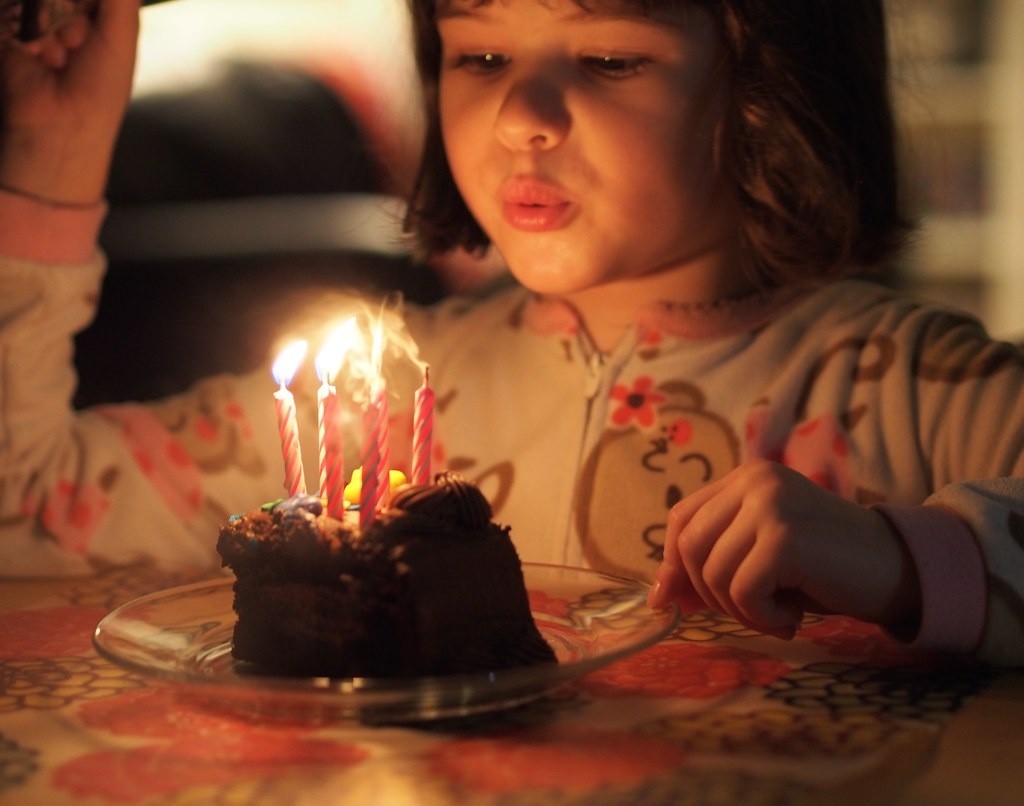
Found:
[217,471,559,679]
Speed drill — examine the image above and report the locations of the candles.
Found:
[412,365,435,485]
[272,377,306,498]
[371,378,390,511]
[319,373,343,521]
[359,385,379,530]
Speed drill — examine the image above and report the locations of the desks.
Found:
[0,562,1024,806]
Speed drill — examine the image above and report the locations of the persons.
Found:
[0,1,1024,675]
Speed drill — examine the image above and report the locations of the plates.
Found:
[94,562,676,718]
